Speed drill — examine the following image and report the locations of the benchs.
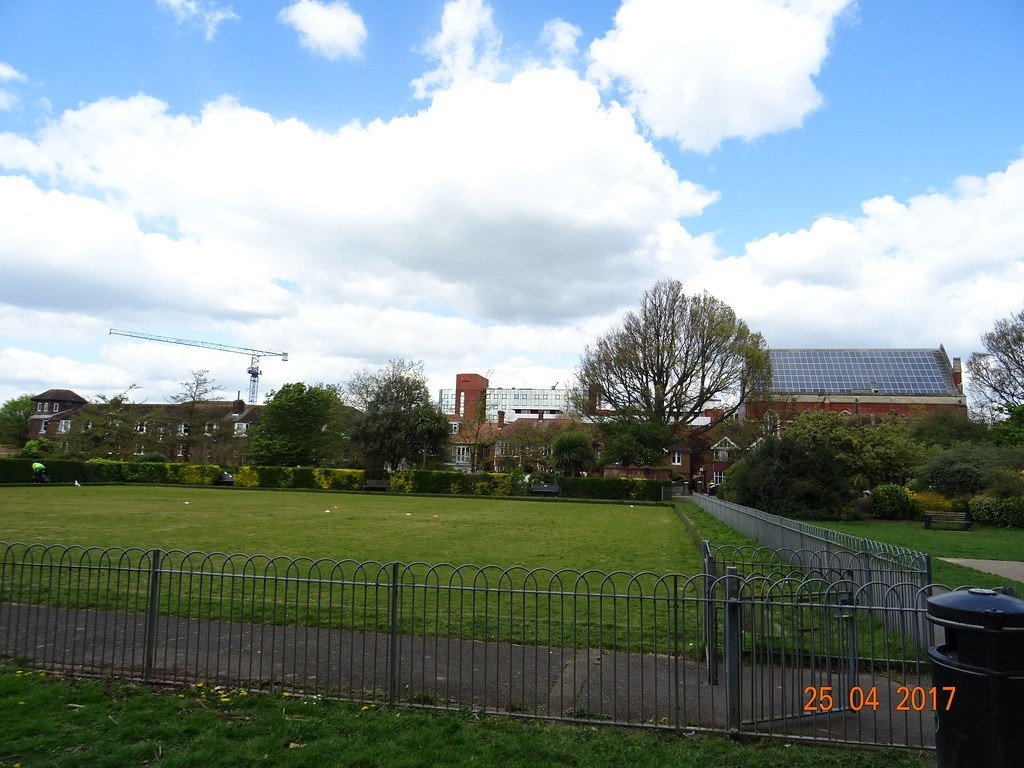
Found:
[924,510,973,531]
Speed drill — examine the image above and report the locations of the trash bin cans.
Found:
[927,588,1024,768]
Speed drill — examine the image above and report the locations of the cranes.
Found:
[108,327,289,403]
